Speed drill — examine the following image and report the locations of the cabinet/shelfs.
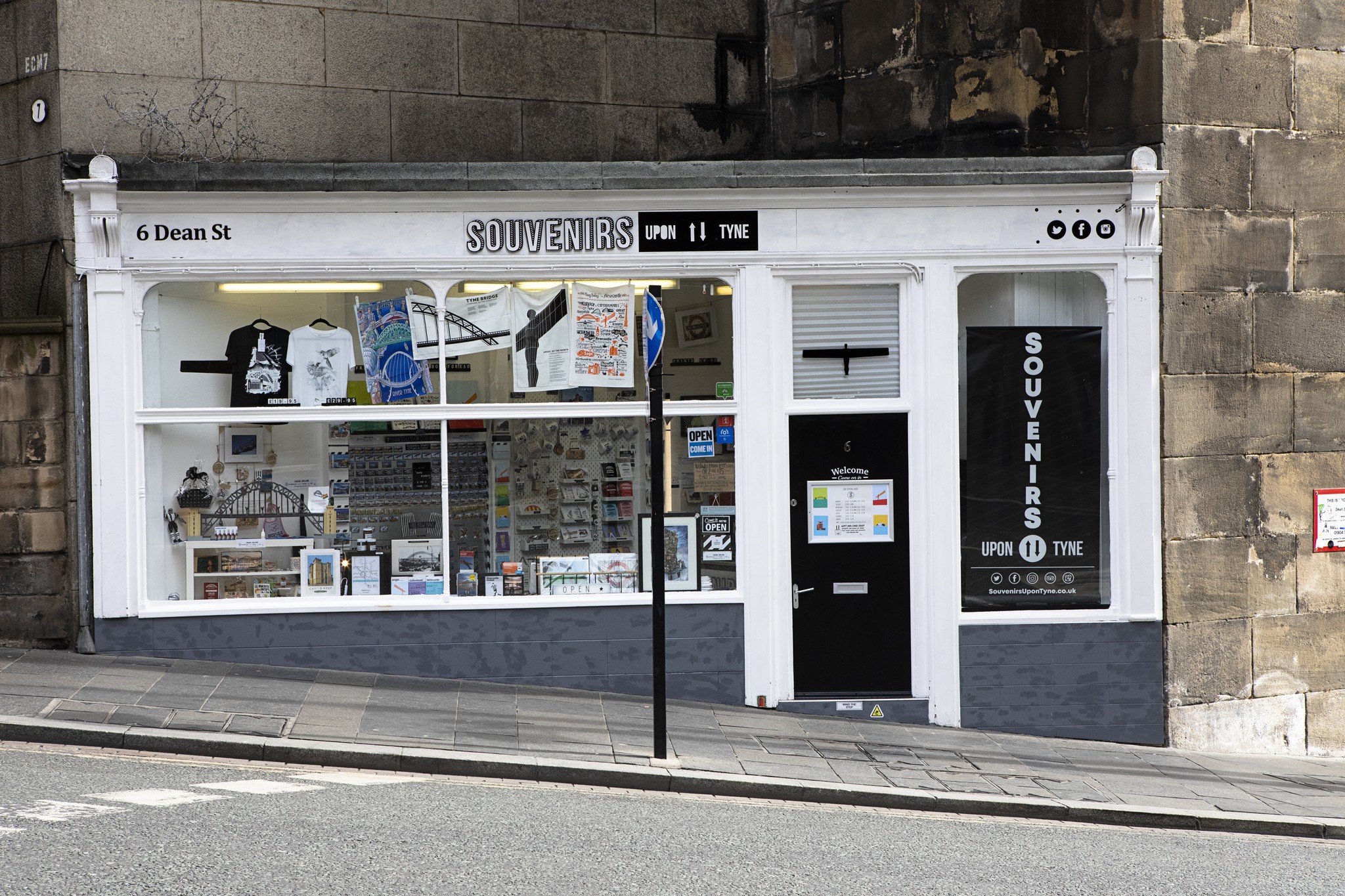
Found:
[516,476,634,550]
[186,538,313,601]
[495,467,511,576]
[348,443,487,524]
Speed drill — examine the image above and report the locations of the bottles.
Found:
[222,530,227,540]
[235,530,238,540]
[226,530,231,540]
[231,530,235,540]
[218,530,222,540]
[215,530,218,541]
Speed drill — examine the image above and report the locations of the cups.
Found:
[597,437,613,454]
[624,422,639,438]
[513,429,528,446]
[609,422,625,439]
[522,419,540,436]
[511,450,527,466]
[539,436,554,453]
[594,420,609,436]
[290,557,300,571]
[527,439,542,455]
[542,418,559,435]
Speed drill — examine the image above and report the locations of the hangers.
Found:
[231,307,291,343]
[291,306,351,340]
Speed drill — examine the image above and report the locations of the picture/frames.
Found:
[391,539,444,575]
[225,427,264,462]
[638,513,701,593]
[685,490,704,502]
[675,306,718,348]
[503,574,524,596]
